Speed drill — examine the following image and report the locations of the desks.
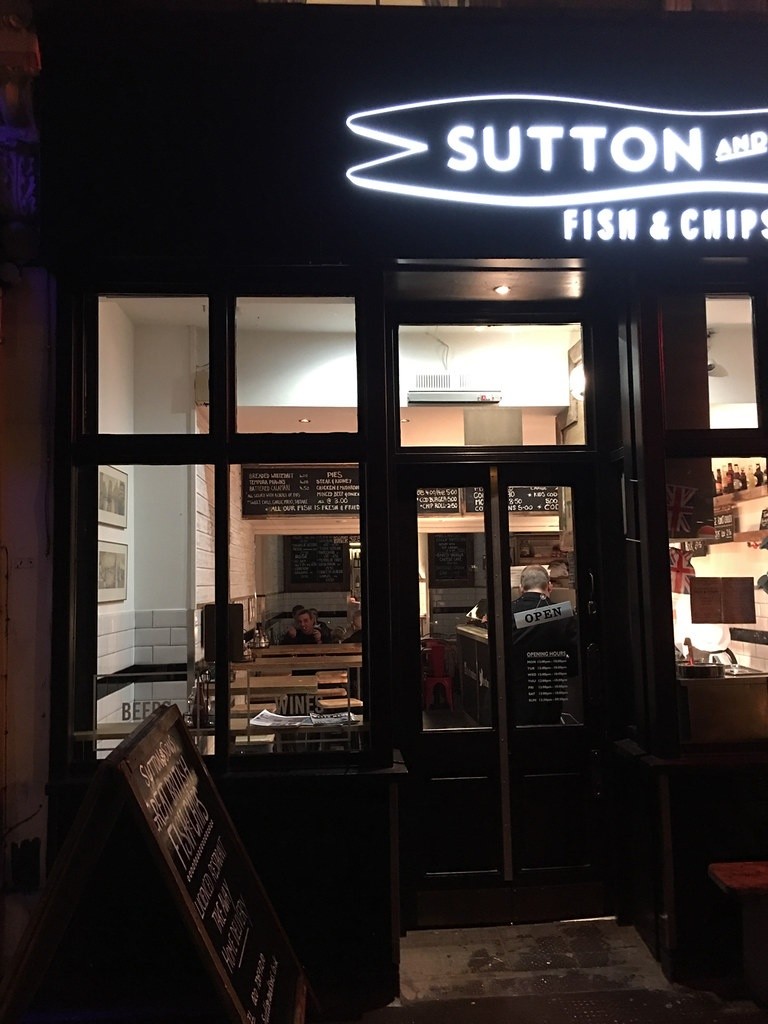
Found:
[198,642,363,756]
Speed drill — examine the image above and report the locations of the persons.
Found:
[278,605,361,698]
[506,563,576,726]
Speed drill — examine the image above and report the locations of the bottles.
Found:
[208,696,215,727]
[715,469,723,496]
[754,464,763,487]
[711,471,717,497]
[193,683,207,728]
[354,552,358,568]
[733,464,741,492]
[727,463,734,493]
[764,465,768,485]
[355,575,359,588]
[253,629,260,648]
[261,632,269,647]
[739,469,747,491]
[358,552,361,568]
[722,465,728,495]
[746,465,755,489]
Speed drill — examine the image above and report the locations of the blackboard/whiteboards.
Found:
[428,532,476,588]
[681,514,736,558]
[283,534,350,592]
[119,701,307,1023]
[416,486,560,513]
[241,467,360,515]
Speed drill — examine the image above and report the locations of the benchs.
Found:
[708,862,768,1010]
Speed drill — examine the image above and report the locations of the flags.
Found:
[665,484,697,594]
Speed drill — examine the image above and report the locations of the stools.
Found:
[423,675,455,714]
